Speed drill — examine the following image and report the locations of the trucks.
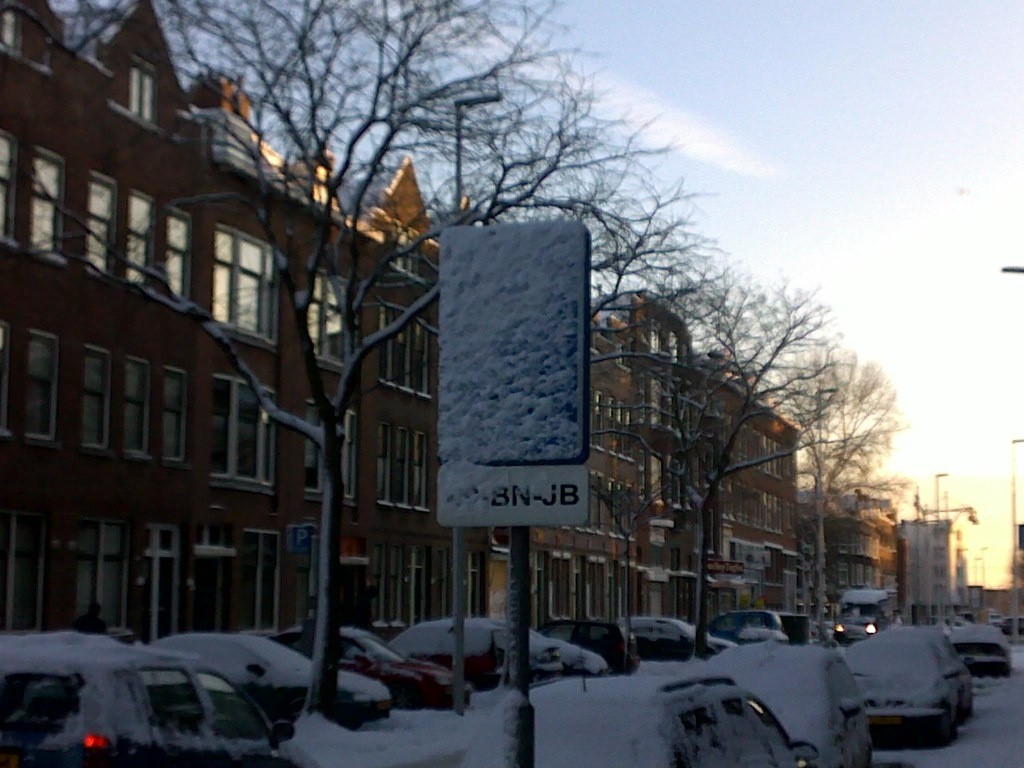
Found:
[832,588,895,644]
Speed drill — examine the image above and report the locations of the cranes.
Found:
[913,482,980,525]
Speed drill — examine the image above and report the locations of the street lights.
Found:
[934,471,950,622]
[451,92,506,714]
[1010,438,1024,646]
[811,386,840,644]
[679,351,728,626]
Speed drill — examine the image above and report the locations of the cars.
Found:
[146,632,396,730]
[530,608,1024,751]
[0,661,297,768]
[454,671,821,768]
[262,622,476,709]
[387,616,563,690]
[706,641,875,768]
